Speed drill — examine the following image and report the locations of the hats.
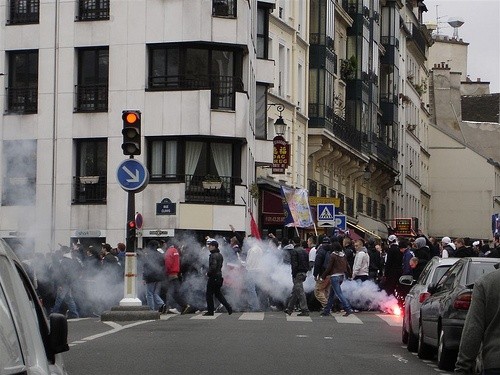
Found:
[322,237,331,243]
[398,241,408,248]
[207,241,218,246]
[388,234,397,243]
[441,237,451,244]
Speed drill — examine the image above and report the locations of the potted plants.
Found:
[339,54,356,80]
[202,174,223,190]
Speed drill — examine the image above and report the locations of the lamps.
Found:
[391,175,402,193]
[364,167,372,180]
[268,104,287,136]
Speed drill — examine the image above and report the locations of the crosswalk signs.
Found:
[317,204,335,221]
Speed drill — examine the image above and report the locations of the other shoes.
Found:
[168,308,181,315]
[282,309,291,316]
[320,313,329,316]
[342,309,353,317]
[202,312,214,316]
[227,306,233,315]
[160,304,167,314]
[297,312,310,316]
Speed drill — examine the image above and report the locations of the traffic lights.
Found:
[121,109,141,156]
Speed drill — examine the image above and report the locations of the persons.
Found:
[368,232,500,311]
[313,236,334,309]
[195,224,371,312]
[141,240,168,315]
[20,236,189,319]
[283,237,310,316]
[454,262,500,375]
[202,241,233,316]
[320,242,353,317]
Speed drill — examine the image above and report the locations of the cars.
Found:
[420,257,500,366]
[1,242,75,375]
[399,255,461,351]
